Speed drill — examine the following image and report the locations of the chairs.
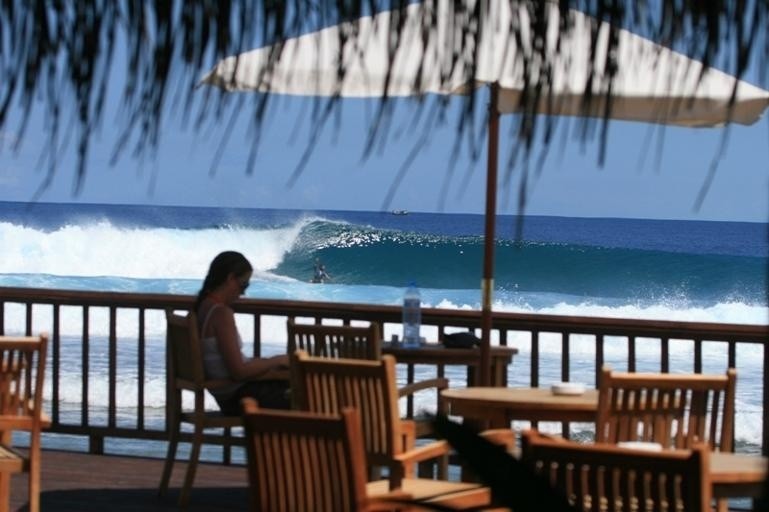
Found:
[1,331,51,512]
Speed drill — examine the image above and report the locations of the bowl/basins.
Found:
[551,381,585,395]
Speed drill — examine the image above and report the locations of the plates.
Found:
[617,441,662,452]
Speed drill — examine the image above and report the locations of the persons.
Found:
[188,251,298,412]
[310,258,328,282]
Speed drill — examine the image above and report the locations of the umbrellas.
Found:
[186,1,768,388]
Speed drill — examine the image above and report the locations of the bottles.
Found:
[390,335,399,346]
[401,280,420,349]
